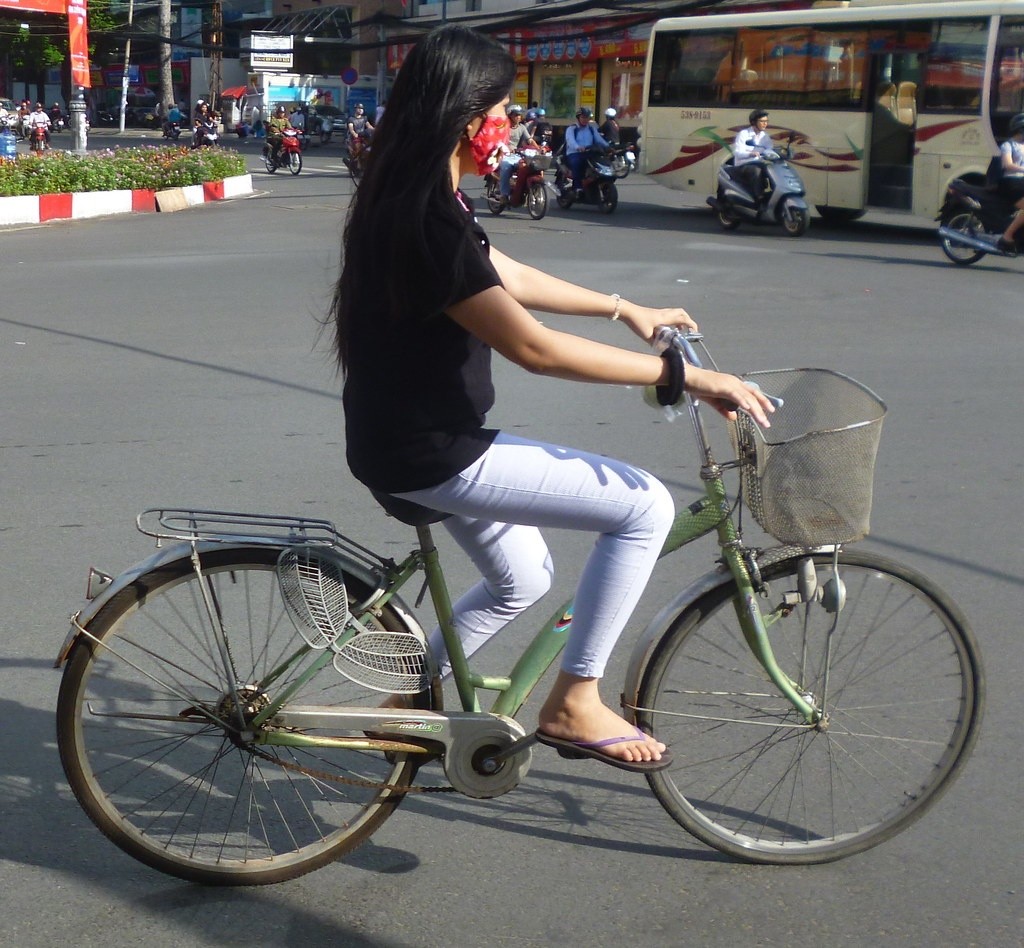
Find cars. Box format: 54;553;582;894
308;105;349;134
0;97;19;129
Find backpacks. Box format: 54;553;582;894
986;140;1015;185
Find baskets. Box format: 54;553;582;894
731;367;889;547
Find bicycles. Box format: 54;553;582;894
51;322;985;887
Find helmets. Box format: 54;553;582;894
168;103;172;108
576;106;592;117
535;109;546;115
605;108;616;116
354;103;364;109
749;109;769;122
1009;113;1024;131
506;105;522;115
36;103;43;108
277;106;286;113
526;109;537;119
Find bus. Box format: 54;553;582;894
638;0;1024;225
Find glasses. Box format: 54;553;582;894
757;119;769;123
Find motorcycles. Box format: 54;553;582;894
480;129;553;220
158;116;182;141
258;114;335;176
545;142;621;216
343;129;376;178
935;178;1024;267
0;114;64;151
613;141;636;179
190;117;219;148
706;130;810;237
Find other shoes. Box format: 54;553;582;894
500;196;510;204
997;237;1016;251
576;189;582;192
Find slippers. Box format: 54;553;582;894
534;724;674;773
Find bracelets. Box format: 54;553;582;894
611;293;621;322
656;347;686;406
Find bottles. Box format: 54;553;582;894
0;128;16;156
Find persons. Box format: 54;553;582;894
500;102;552;206
589;108;620;145
0;95;63;150
734;109;793;210
151;99;185;137
334;27;776;772
566;106;615;195
999;114;1024;252
235;118;270;139
345;100;388;162
193;99;222;149
269;105;334;162
